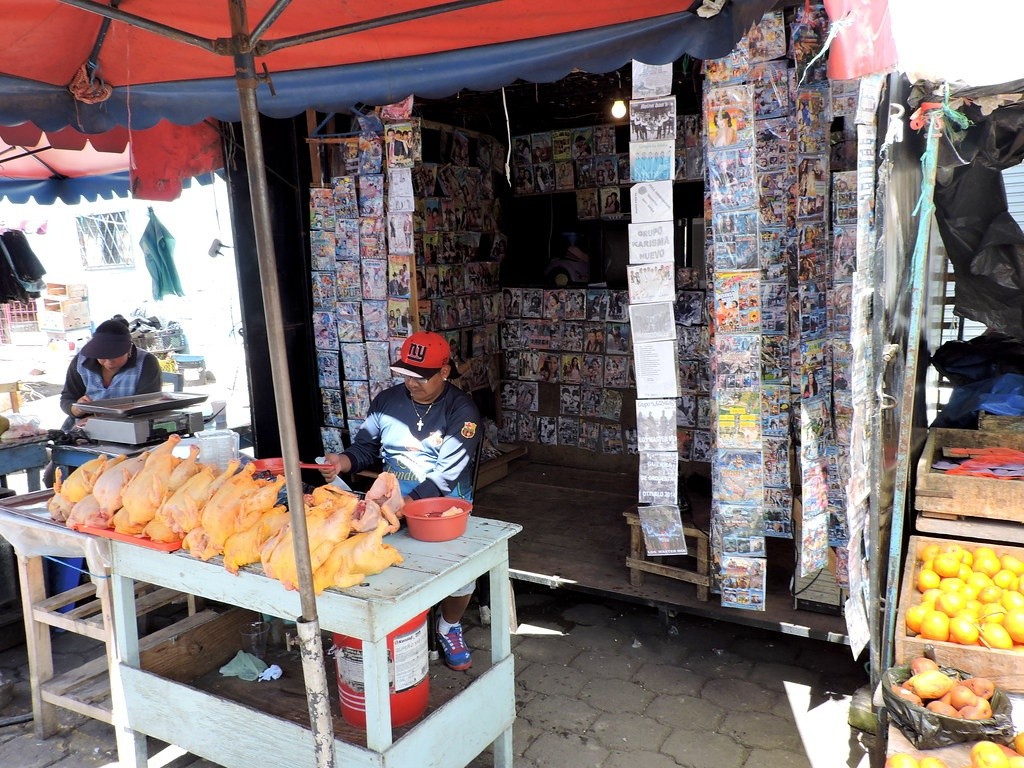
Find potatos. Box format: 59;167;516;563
892;657;994;721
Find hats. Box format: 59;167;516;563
390;331;450;379
834;228;842;236
804;101;809;106
81;333;131;359
749;562;763;572
781;404;788;409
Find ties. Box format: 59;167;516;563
388;144;393;166
399;319;400;324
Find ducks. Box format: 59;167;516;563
47;434;404;595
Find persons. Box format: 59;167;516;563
418;137;501;461
631;20;881;609
498;288;638;458
309;125;415;463
318;332;480;670
510;127;630;220
43;315;162;488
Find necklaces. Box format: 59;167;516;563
411;392;432;432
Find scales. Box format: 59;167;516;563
70;391;210;450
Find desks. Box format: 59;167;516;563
50;413;255;484
0;478;524;768
0;434;50;501
623;475;710;602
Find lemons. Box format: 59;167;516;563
886;732;1024;767
906;544;1024;653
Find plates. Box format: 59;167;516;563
172;438;200;460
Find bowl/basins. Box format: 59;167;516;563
882;665;1012;740
401;497;473;541
172;354;205;362
194;429;239;477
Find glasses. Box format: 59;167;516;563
400;369;440;383
515;143;521;147
576;144;585;149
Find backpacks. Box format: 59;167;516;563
521;139;530;154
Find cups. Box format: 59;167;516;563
238;621;270;660
212;400;226;422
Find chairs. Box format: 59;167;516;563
429;414;491;662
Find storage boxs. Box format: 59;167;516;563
135;333;187;356
39;282;93;358
895;426;1024;695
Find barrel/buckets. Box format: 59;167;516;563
333;606;429;728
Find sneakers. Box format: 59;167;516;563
436;616;472;670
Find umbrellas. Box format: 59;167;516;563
0;1;779;768
